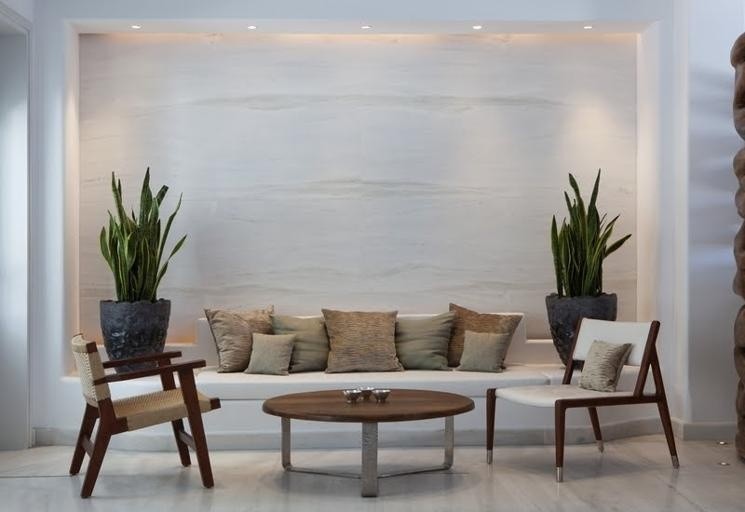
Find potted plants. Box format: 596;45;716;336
99;167;187;372
545;167;633;370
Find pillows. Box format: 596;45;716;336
321;308;404;373
395;310;457;371
455;330;509;372
202;304;274;372
578;339;633;392
270;314;328;373
243;332;295;375
448;302;522;372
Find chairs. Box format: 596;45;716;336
486;315;680;481
69;332;221;498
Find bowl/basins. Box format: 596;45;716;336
358;387;373;400
373;389;390;402
342;389;361;401
